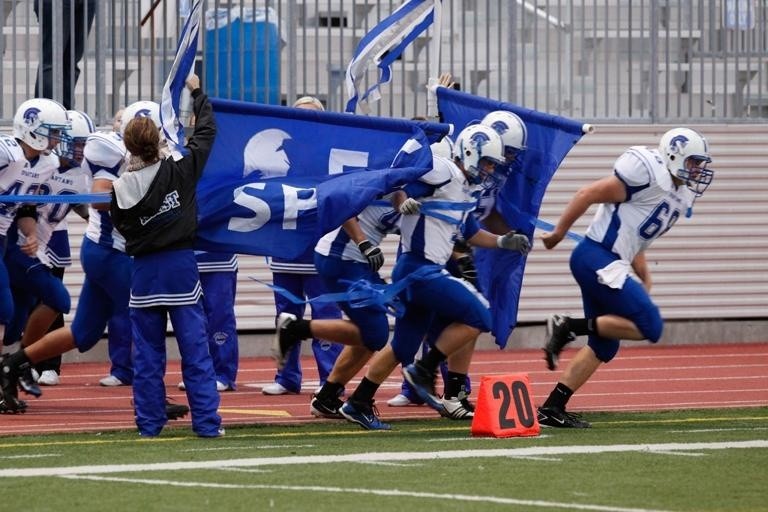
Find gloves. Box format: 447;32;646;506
357;239;384;273
456;256;478;286
498;231;531;255
401;199;421;215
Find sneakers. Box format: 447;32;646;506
262;383;301;395
541;314;576;371
178;382;185;391
98;376;123;386
218;429;225;435
314;385;344;397
537;407;592;428
400;359;445;411
0;353;28;414
216;380;236;391
270;312;297;371
441;395;475;420
31;369;40;382
388;394;425;406
17;368;42;397
38;371;60;386
339;397;391;430
131;396;189;421
310;393;345;418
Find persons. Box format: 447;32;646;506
0;98;74;414
174;251;242;395
0;100;162;410
29;311;66;387
437;110;530;424
10;109;96;401
98;311;135;387
535;127;715;428
109;72;228;439
262;97;346;398
272;133;455;421
336;123;493;431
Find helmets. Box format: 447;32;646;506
453;125;506;190
480;111;530;178
431;136;454;162
658;128;714;193
120;101;166;142
13;98;73;157
66;110;95;144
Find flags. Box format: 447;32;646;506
435;85;592;350
192;92;455;264
